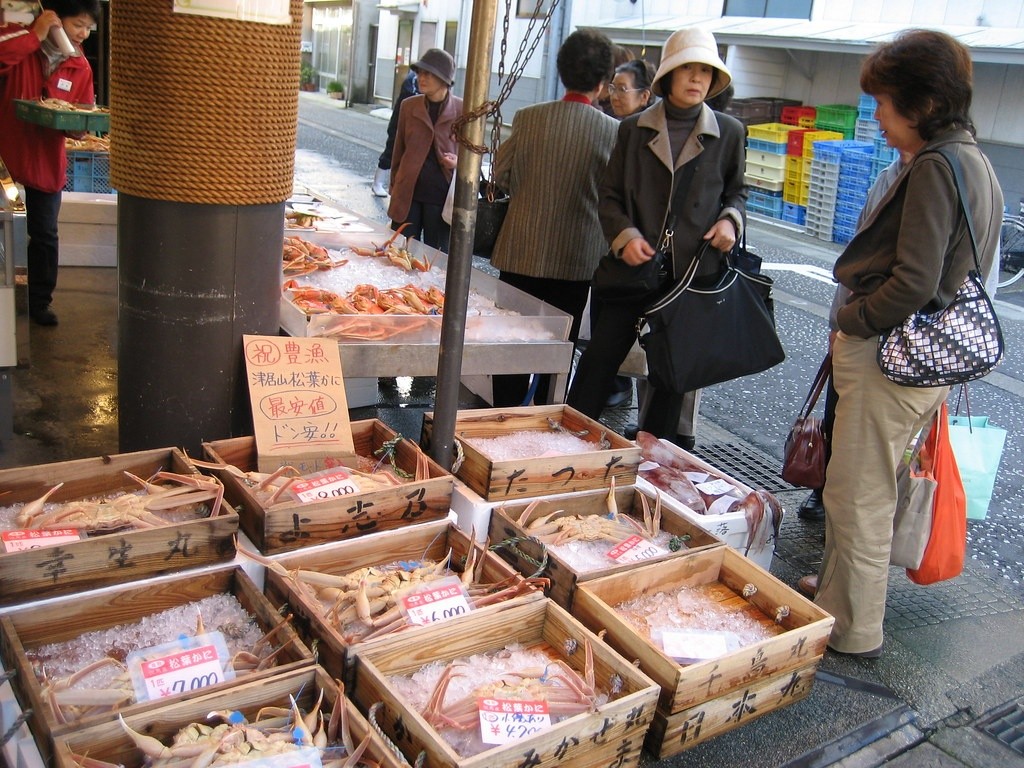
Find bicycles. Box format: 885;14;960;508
995;199;1024;289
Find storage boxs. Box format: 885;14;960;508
17;98;110;131
0;404;836;767
724;94;900;246
0;150;118;267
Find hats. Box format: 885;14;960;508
650;28;732;99
409;49;455;86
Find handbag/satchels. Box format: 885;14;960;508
645;216;785;395
729;206;762;277
472;179;509;259
875;149;1004;387
948;382;1006;521
781;354;832;488
442;169;483;226
888;403;965;584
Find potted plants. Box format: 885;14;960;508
326;81;343;98
300;60;315;91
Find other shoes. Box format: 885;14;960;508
624;423;695;451
603;386;633;409
29;311;57;326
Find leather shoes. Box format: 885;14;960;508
798;492;825;518
825;637;884;657
798;575;818;597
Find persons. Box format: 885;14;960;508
490;25;748;446
370;49;465;395
0;0;100;328
799;33;1005;663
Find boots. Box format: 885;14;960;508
372;166;388;197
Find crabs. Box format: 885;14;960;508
41;522;597;768
518;469;664;548
34;94;111;153
15;440;437;538
281;210;447;341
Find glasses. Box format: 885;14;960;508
608;84;643;97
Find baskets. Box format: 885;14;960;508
746;122;805;154
804;139;874;246
745;190;783;221
854;96;882;142
722;97;802;156
